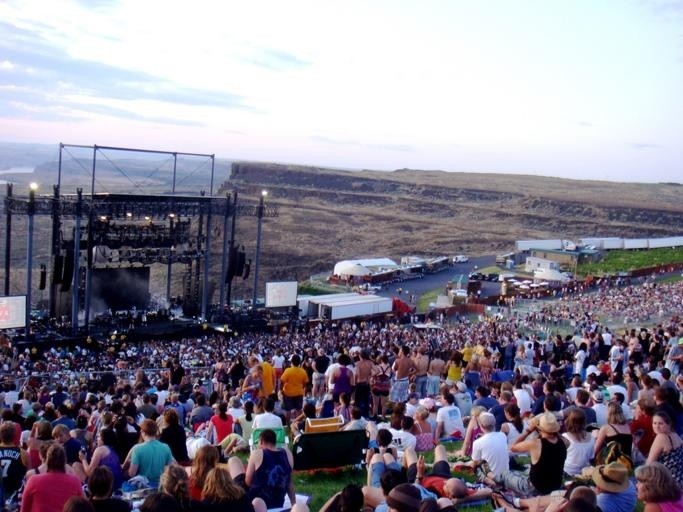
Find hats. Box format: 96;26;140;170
479;410;633;492
385;483;422;512
139;419;157;432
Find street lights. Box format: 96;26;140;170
24;181;40;338
250;190;267;313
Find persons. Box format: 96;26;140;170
495;269;681;326
0;303;682;512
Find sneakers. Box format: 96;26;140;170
473;459;494;484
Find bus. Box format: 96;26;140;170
495;252;515;264
422;256;449;274
362;265;424;289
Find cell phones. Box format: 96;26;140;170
81;445;86;455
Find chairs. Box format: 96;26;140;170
304;417;347;432
248;425;288;456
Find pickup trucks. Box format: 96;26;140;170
358;283;382;293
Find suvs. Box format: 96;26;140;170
453;255;468;263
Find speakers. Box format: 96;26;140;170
53;256;64;285
61;245;75;292
40;271;46;290
225;247;237;283
237;252;245;276
243;264;250;280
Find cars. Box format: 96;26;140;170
452;289;467;296
468;272;486;281
